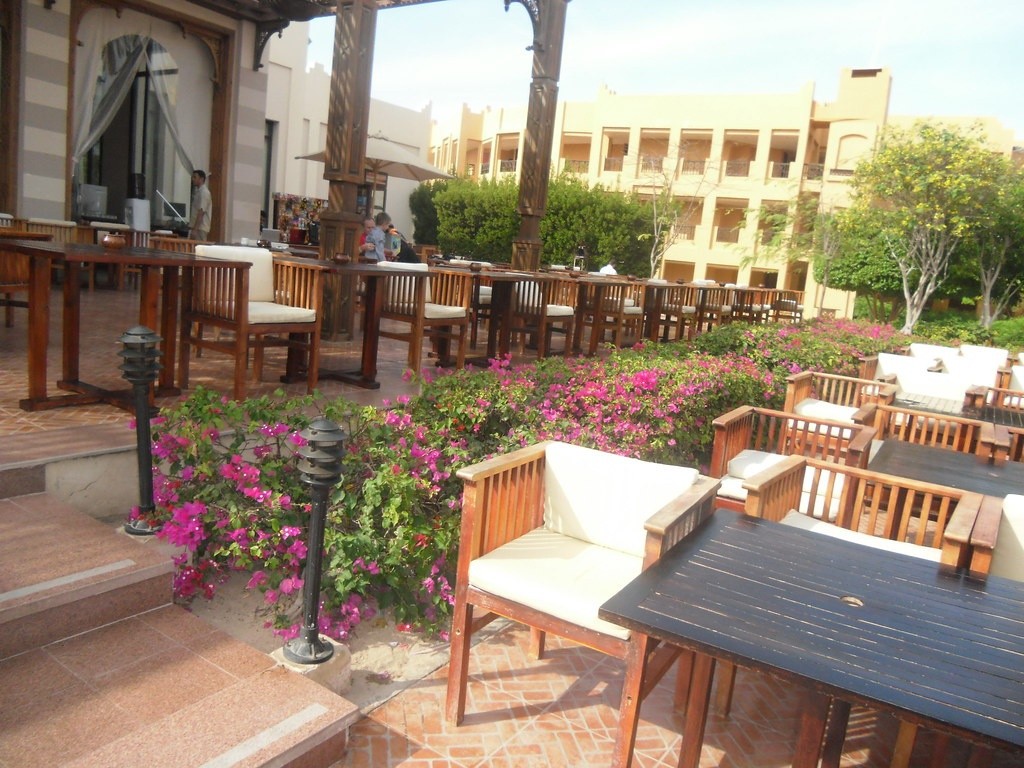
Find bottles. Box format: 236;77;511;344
126;174;145;200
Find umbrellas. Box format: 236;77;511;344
295;139;455;215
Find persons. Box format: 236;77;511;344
260;210;265;231
600;258;618;275
358;212;398;262
188;170;213;241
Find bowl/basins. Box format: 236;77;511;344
103;234;126;249
569;271;580;278
676;279;684;284
334;253;349;265
469;262;482;272
719;282;726;287
627;274;636;282
257;239;271;247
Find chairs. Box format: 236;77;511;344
0;212;808;402
446;342;1024;767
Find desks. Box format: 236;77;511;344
432;267;536;371
980;408;1024;462
548;272;634;359
276;256;441;390
863;438;1024;544
698;286;724;332
735;288;760;323
638;281;688;342
597;509;1024;768
0;236;253;420
